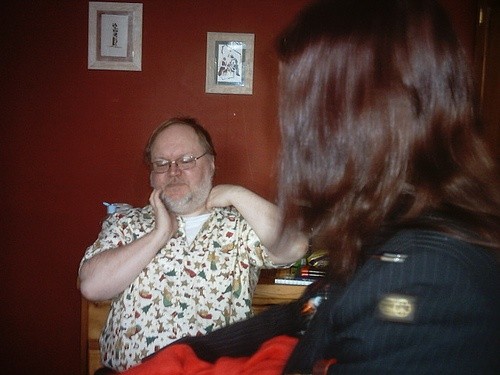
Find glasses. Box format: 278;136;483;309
150;150;209;174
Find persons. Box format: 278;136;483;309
120;1;500;375
78;115;309;375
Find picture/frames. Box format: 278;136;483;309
205;32;254;95
88;2;143;71
